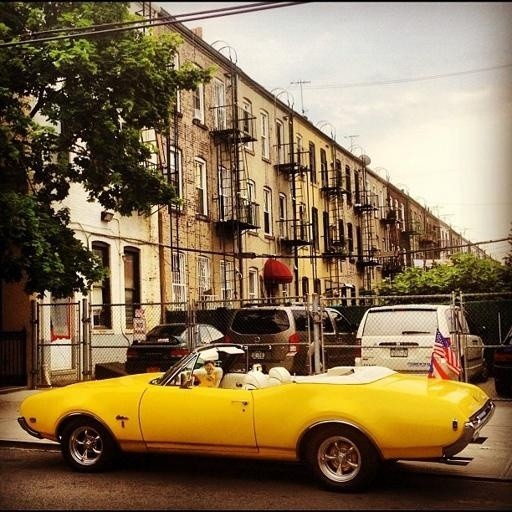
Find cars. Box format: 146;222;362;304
16;343;496;493
489;328;512;397
126;323;225;372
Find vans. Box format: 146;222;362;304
222;301;356;377
353;304;490;384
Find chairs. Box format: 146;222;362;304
269;366;293;385
243;371;269;389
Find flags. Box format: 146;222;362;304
428;328;463;380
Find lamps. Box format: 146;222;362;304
101;212;114;222
